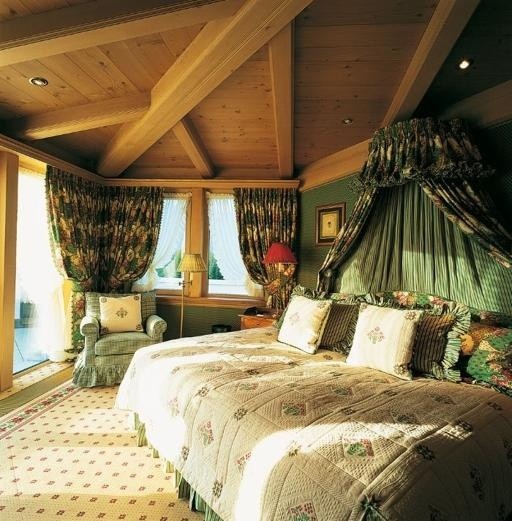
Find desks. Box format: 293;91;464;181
152;289;268;310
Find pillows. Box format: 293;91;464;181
366;292;470;383
378;291;512;399
276;284;359;356
346;302;424;380
99;294;145;335
276;295;333;355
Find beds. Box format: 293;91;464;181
109;291;512;521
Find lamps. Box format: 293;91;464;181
177;254;208;339
262;242;298;319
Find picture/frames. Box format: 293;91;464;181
315;202;345;246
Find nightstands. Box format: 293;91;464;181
237;312;276;331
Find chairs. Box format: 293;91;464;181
72;294;167;388
14;302;37;362
